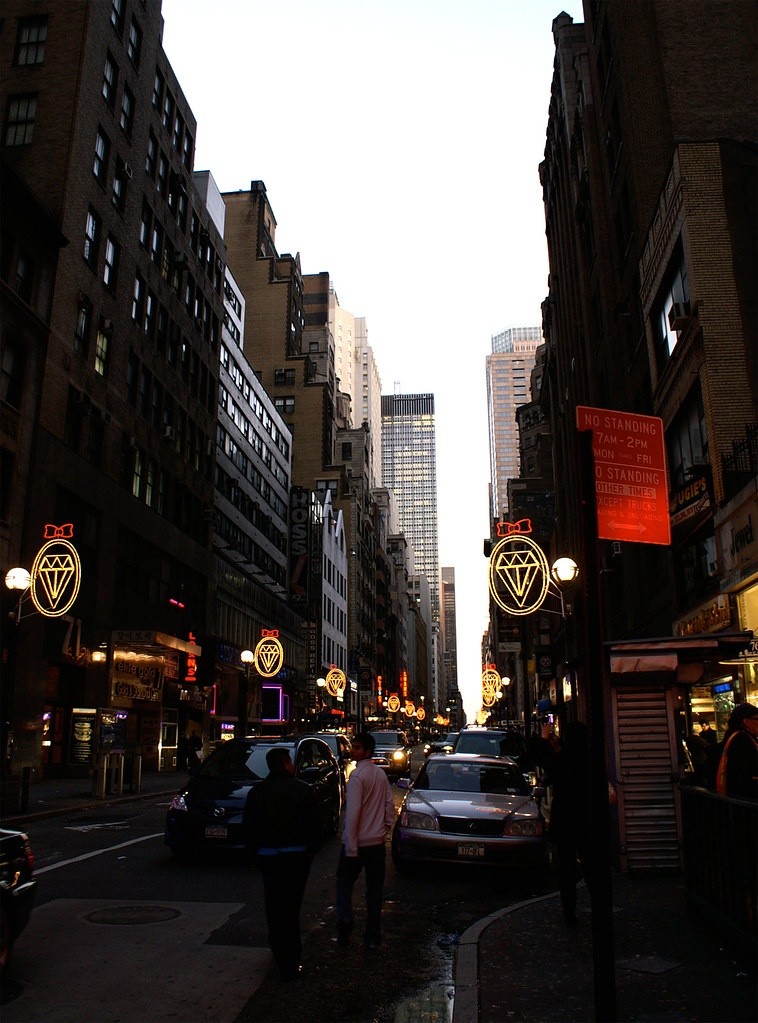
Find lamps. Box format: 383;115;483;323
316;678;326;689
240;650;255;678
446;708;451;711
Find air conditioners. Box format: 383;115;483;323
164;425;175;441
667;302;689;330
122;161;133;180
196;318;203;330
105;319;115;334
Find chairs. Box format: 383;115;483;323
478;768;516;794
430;764;462;790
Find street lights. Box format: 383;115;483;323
549;554;583;746
240;649;255;698
317;677;325;705
4;565;32;686
382;696;495;725
501;676;511;724
496;691;504;722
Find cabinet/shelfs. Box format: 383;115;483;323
139;706;180;774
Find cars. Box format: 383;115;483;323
300;724;538;821
0;827;37;973
162;734;342;868
389;751;543;883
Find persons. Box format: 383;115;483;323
533;718;606;926
336;733;395;948
243;748;333;983
678;703;758;928
178;729;204;767
